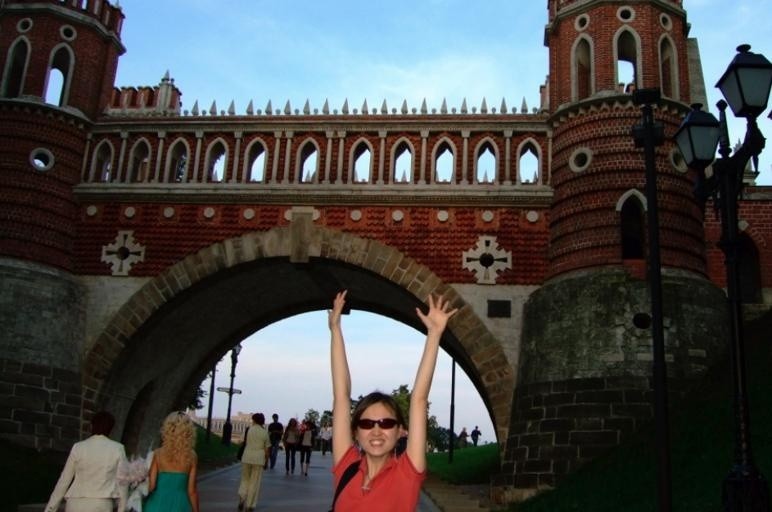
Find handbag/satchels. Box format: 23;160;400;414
237;428;249;460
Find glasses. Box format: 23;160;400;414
358;418;397;429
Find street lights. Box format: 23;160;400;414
224;342;242;444
670;45;772;512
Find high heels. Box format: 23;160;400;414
239;503;256;512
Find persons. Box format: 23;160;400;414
318;290;458;512
237;414;332;512
458;426;481;447
45;410;199;512
